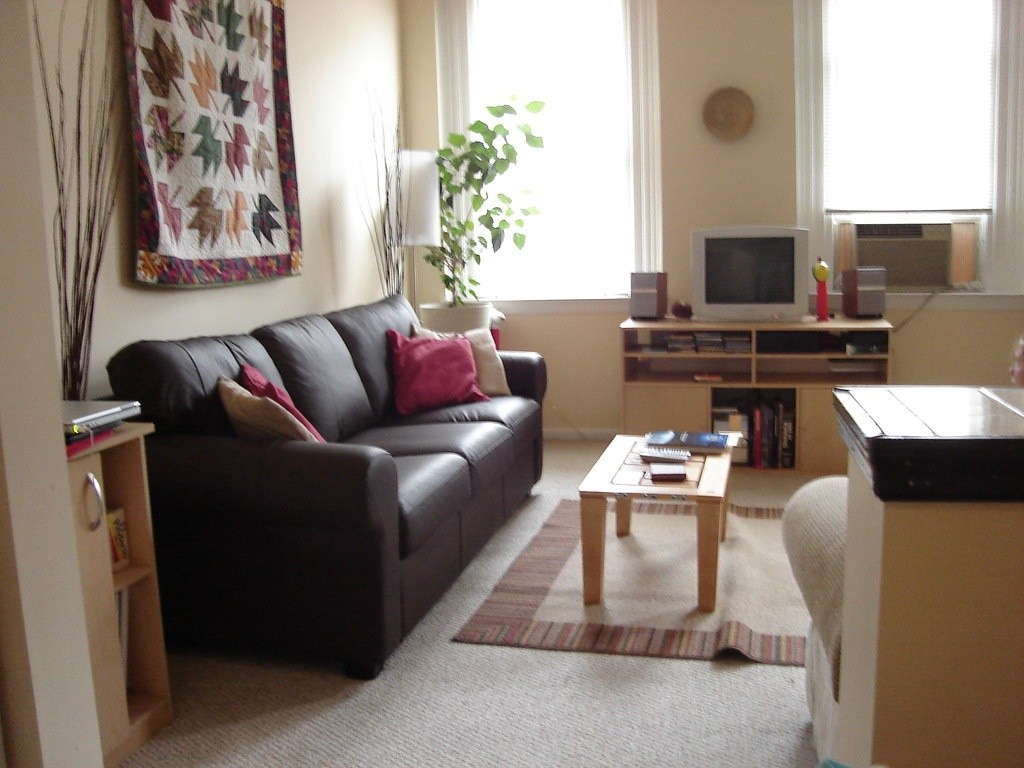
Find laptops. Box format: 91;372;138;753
62;400;142;434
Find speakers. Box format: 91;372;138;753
630;272;668;319
842;267;887;318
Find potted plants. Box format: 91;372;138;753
418;99;547;336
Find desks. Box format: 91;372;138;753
577;431;736;611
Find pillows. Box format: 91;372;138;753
410;323;512;397
218;375;317;444
240;359;330;444
385;325;490;419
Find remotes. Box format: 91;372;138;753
638;446;691;464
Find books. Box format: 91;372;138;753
112;590;130;687
639;429;728;482
712;392;795;469
724;336;750;353
663;334;695;352
694;332;724;352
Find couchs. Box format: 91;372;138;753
105;289;548;684
779;470;849;761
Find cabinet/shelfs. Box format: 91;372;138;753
831;382;1023;768
67;417;176;768
616;309;894;471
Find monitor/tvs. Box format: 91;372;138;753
689;226;811;321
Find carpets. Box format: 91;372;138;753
455;497;809;668
